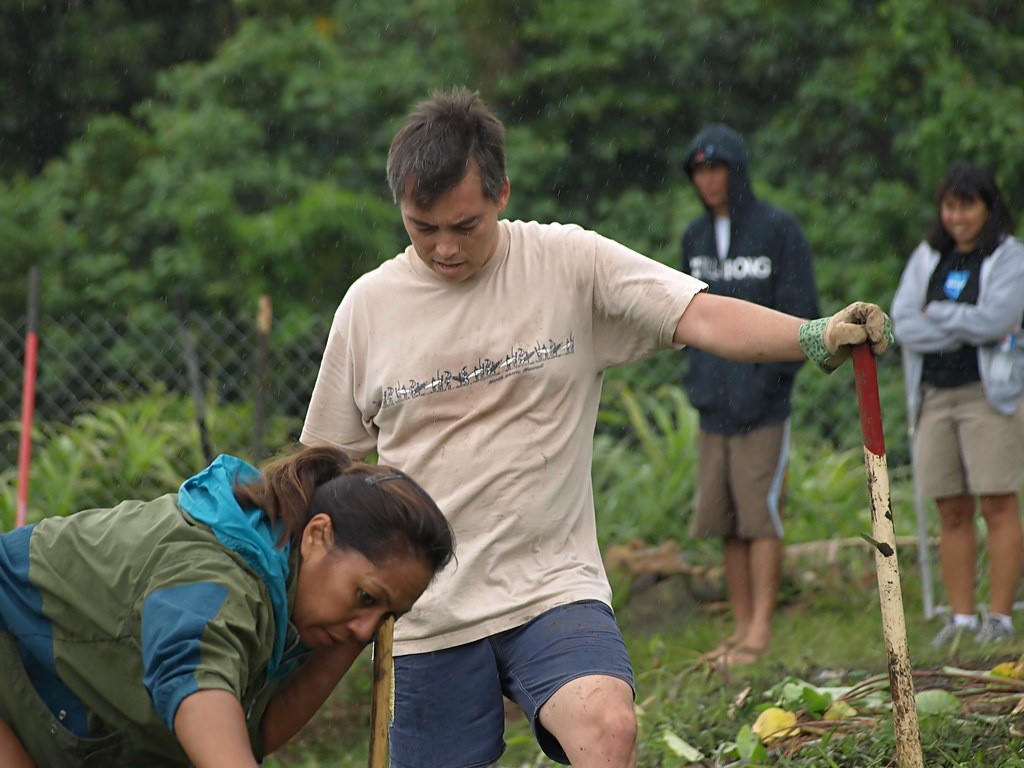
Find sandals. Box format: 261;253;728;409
696;638;774;671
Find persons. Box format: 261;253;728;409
680;121;820;670
0;447;454;768
298;91;891;768
889;164;1024;647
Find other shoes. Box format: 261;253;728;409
972;613;1015;648
930;613;982;651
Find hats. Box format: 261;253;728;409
690;150;727;166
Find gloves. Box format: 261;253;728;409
798;301;894;376
